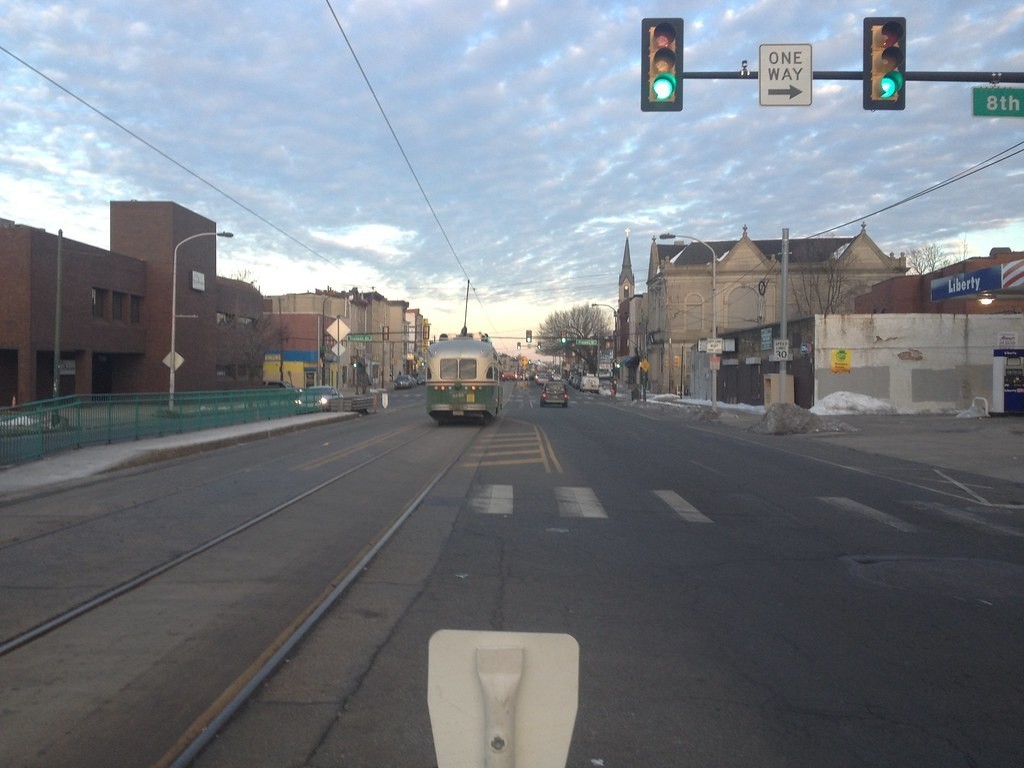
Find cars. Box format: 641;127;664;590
294;386;344;413
540;380;568;407
501;370;560;383
394;374;425;389
264;381;303;398
568;374;599;394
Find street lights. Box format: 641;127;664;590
659;233;718;414
591;302;616;330
322;291;354;386
167;231;233;410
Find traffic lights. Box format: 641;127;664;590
538;341;542;350
560;338;568;344
862;17;905;110
642;18;683;111
516;342;521;349
525;329;532;343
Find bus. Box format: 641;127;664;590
425;280;503;423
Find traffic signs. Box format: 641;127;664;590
758;43;812;106
